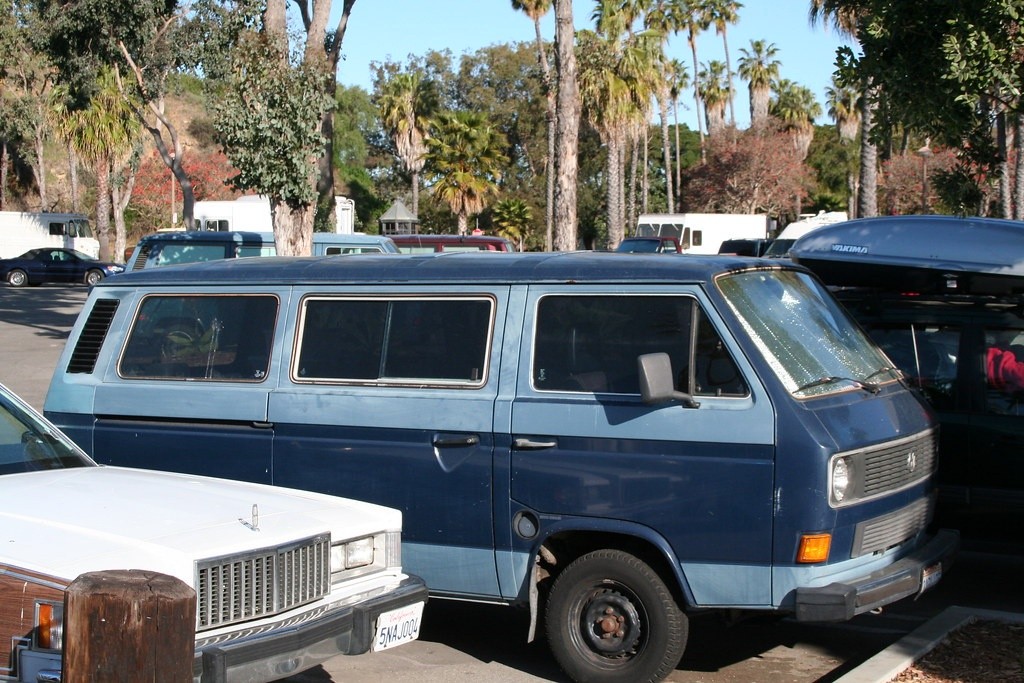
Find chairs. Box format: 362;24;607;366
54;255;60;260
48;255;52;260
568;366;632;392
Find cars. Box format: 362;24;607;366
0;246;132;289
781;216;1023;562
0;379;432;683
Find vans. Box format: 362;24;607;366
118;229;404;276
1;210;101;258
32;251;960;683
344;237;518;272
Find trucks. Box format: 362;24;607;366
760;209;849;261
614;213;773;253
